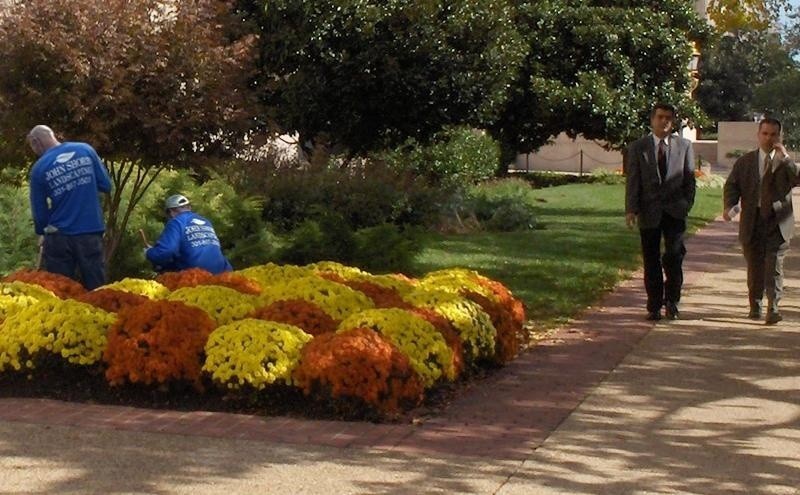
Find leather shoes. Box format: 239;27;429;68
749;309;782;323
646;301;679;320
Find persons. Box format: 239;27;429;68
27;123;113;292
142;192;233;277
623;101;697;320
723;116;799;324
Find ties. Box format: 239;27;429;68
760;154;772;221
658;140;667;182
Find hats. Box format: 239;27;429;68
165;194;189;209
